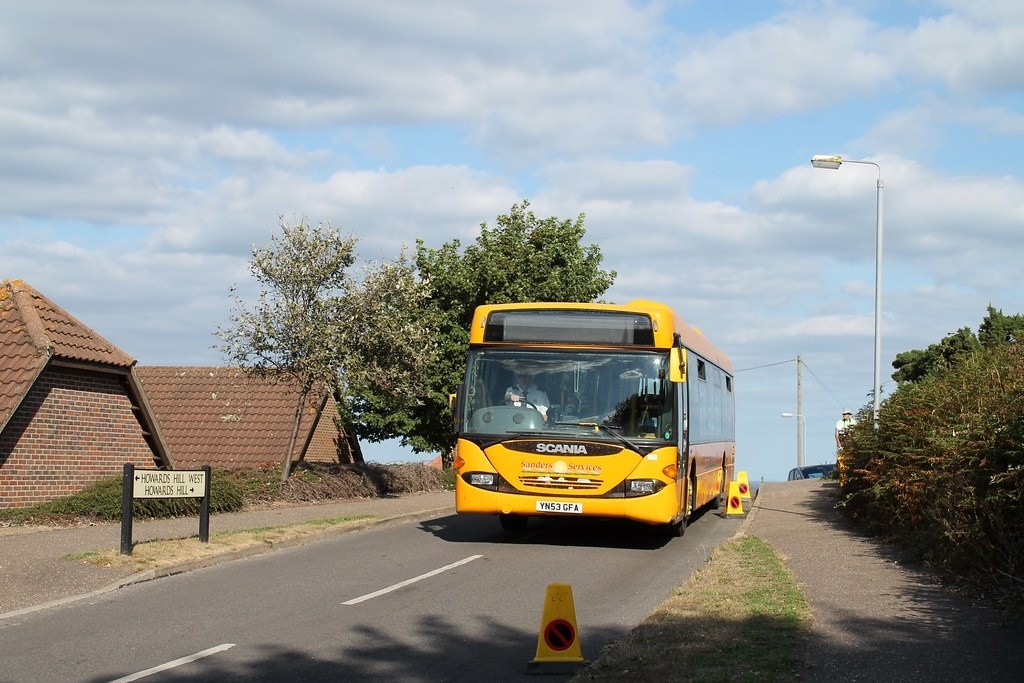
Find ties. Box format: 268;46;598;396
521;391;529;408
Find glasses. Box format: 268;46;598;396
517;374;527;379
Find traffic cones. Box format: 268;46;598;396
718;480;748;520
524;582;594;678
736;470;752;502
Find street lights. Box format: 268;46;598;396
783;411;807;468
810;150;885;435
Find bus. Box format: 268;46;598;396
445;297;735;537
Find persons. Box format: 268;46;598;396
505;366;550;423
547;372;655;428
835;408;859;486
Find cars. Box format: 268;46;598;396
788;464;839;482
